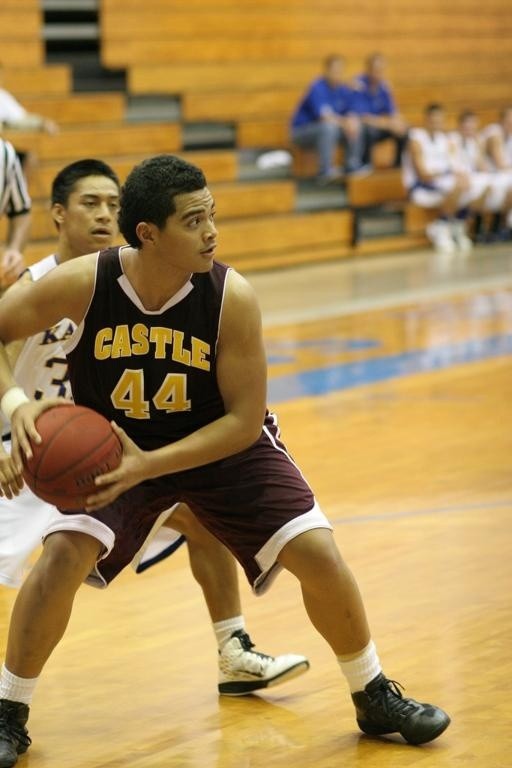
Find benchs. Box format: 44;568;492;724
0;0;512;291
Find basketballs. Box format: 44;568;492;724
18;404;121;511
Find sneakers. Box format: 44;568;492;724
217;633;311;697
0;698;30;767
350;671;450;746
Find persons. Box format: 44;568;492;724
1;136;34;281
289;51;511;254
0;155;451;768
1;160;309;696
